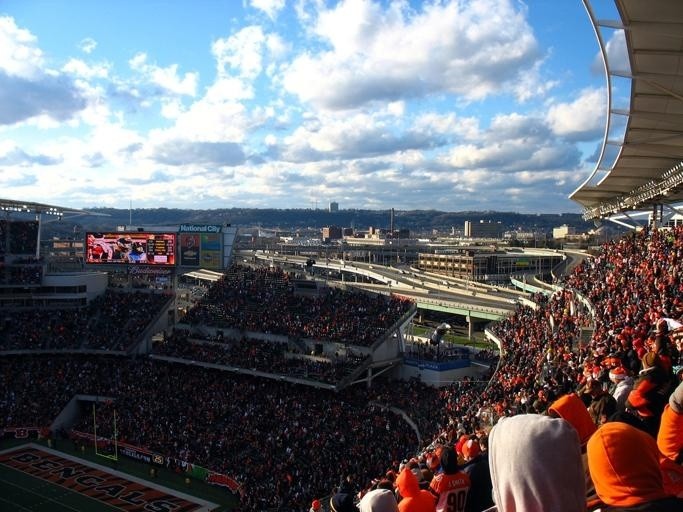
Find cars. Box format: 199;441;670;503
470;282;478;287
492;287;499;292
439;281;459;288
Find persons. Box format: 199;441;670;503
0;219;683;512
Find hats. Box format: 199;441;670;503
312;500;320;508
643;352;661;368
608;367;625;380
462;440;480;457
329;493;354;512
455;437;468;452
